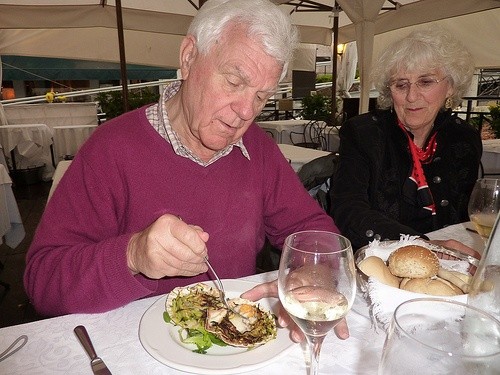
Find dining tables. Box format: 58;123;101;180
0;223;485;375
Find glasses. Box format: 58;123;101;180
387;76;447;94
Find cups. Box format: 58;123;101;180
460;214;500;358
377;299;500;375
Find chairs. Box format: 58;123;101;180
266;111;347;209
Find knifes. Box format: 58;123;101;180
74;326;112;375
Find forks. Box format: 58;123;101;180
178;216;238;315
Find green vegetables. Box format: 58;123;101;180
162;286;276;354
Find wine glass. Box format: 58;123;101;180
278;230;357;374
468;178;500;244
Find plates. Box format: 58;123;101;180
139;278;299;374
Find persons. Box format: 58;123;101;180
23;0;349;344
333;30;483;259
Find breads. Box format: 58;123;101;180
357;244;494;297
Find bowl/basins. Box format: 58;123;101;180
353;239;480;297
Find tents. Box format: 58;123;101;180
0;0;500;112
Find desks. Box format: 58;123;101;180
0;124;56;171
276;141;341;198
52;125;99;161
257;120;326;145
